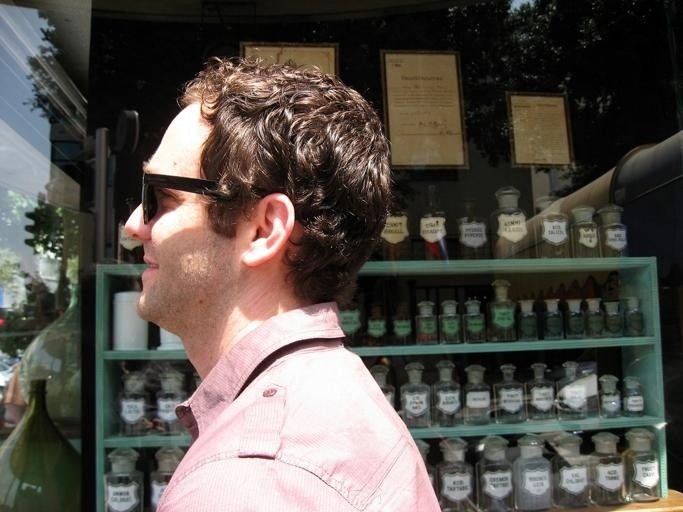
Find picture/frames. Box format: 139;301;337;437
379;49;470;170
238;40;340;82
504;90;577;170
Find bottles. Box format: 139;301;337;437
384;184;627;260
415;429;660;512
371;357;650;424
116;363;192;437
341;277;650;350
103;446;183;509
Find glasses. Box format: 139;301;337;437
142;171;300;224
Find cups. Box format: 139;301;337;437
111;291;148;350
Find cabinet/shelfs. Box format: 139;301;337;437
94;255;669;512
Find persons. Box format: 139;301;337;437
0;272;75;430
122;52;440;511
